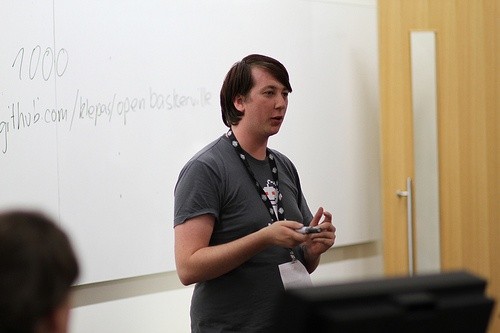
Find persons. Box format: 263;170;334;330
0;211;79;333
172;54;335;333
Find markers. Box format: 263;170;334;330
293;226;321;235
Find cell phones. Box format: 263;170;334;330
297;226;320;234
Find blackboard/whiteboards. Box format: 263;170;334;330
0;0;379;294
